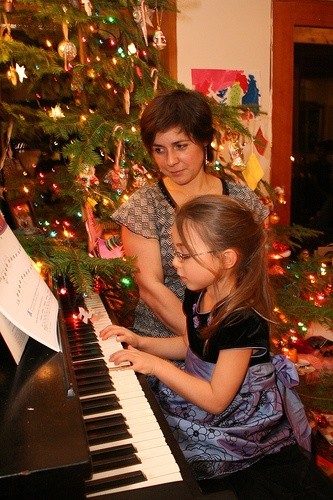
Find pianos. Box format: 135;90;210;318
0;242;206;500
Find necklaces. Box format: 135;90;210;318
166;177;212;199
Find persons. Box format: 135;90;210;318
100;195;313;481
110;91;272;388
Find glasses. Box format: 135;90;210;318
169;246;220;262
